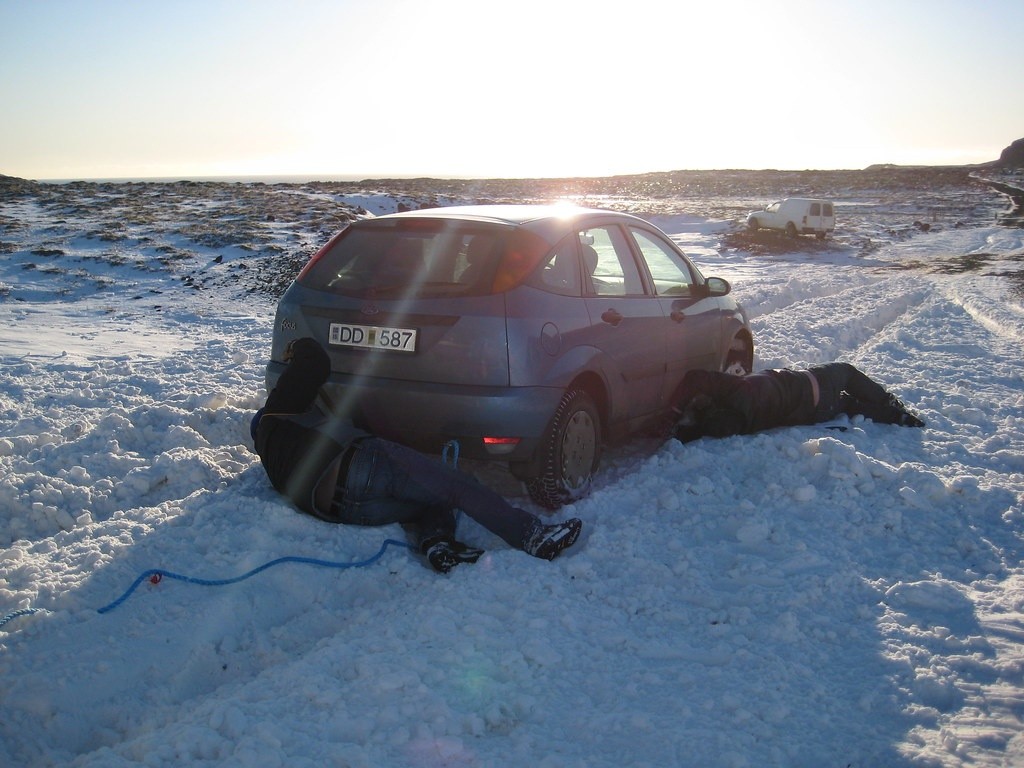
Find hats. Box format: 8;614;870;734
250;409;266;439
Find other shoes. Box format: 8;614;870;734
905;416;924;428
889;392;906;412
523;518;582;559
424;539;484;573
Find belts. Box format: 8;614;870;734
332;437;361;516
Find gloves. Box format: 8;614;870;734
661;407;677;435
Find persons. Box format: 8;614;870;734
663;363;925;444
250;337;581;573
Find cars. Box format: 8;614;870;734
264;201;754;511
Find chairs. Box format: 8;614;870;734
544;244;598;293
370;240;432;279
459;236;495;284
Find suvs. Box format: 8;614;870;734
746;196;836;241
